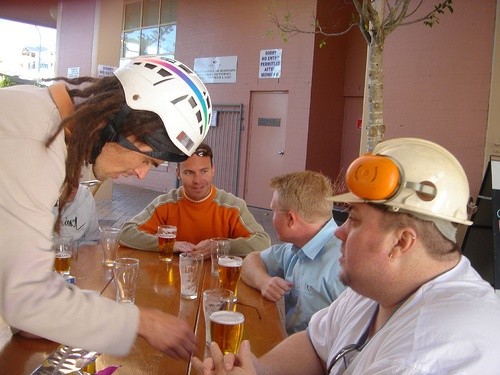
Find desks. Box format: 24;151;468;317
0;245;285;375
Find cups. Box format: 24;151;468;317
113;258;140;304
218;255;242;303
202;288;234;347
209;310;245;355
179;252;203;300
100;227;123;266
158;225;177;262
209;237;231;277
54;236;78;283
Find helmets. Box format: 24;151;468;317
325;137;473;226
113;54;213;157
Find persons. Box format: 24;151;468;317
116;143;271;262
192;136;500;375
53;185;102;246
240;170;348;336
0;57;213;362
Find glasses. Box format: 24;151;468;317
191;149;212;166
327;343;360;375
